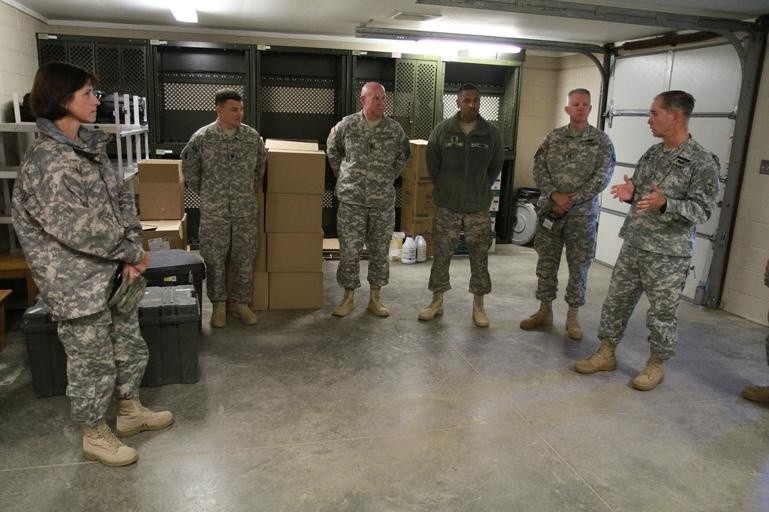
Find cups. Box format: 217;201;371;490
161;288;178;304
138;290;153;307
160;240;171;257
148;238;162;254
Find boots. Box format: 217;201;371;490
521;301;555;330
565;305;583;340
211;301;226;328
230;302;258;324
333;289;356;317
418;292;443;320
742;385;769;404
574;340;617;373
367;288;390;316
633;354;663;390
115;397;174;437
472;294;489;326
83;419;138;467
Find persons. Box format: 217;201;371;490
520;88;616;341
572;90;721;392
10;62;174;468
181;90;267;329
326;81;412;319
418;84;503;327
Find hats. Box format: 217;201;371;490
109;271;148;323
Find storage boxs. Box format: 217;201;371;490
145;249;206;332
227;192;324;312
401;178;437;256
265;140;319;151
401;139;432;183
138;174;185;220
19;284;202;399
267;149;325;194
137;160;183;183
139;213;190;252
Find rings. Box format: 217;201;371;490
135;272;139;277
